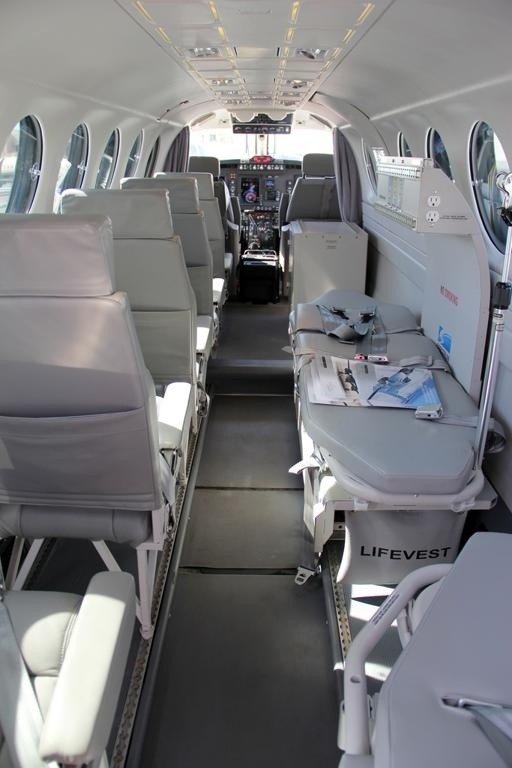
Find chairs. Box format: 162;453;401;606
277;153;344;297
0;155;243;638
1;570;143;768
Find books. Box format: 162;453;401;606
308;349;444;411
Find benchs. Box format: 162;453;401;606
290;289;497;590
